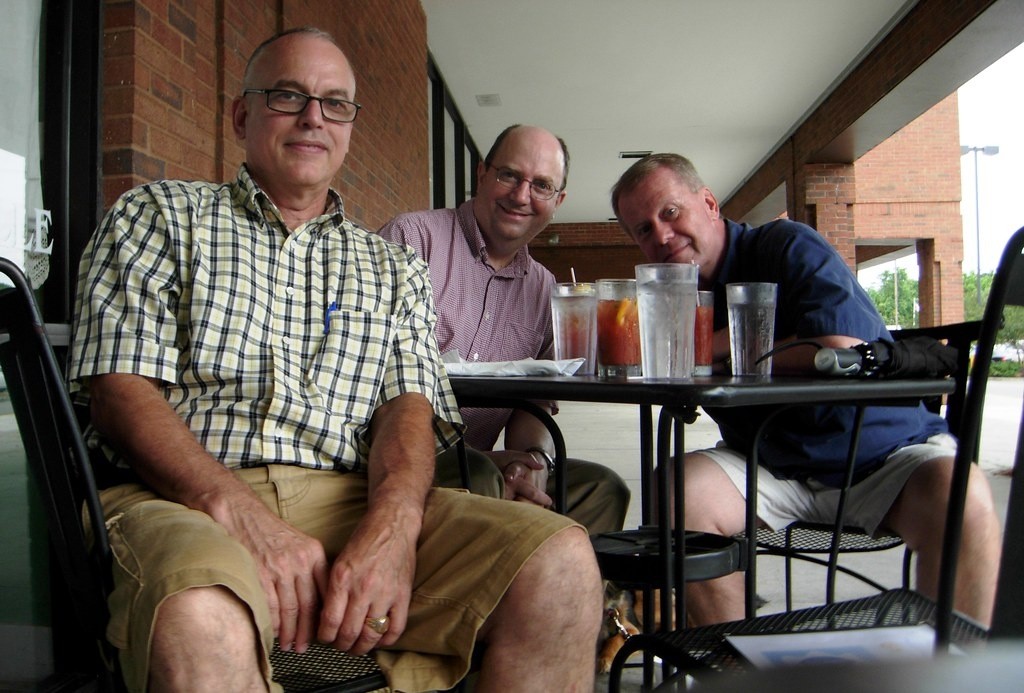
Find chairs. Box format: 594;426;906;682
0;258;388;693
608;228;1024;693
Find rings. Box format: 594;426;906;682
365;617;390;634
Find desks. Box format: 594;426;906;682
449;372;957;693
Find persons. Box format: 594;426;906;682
375;124;630;548
613;152;1000;631
65;26;605;692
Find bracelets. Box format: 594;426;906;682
527;446;556;476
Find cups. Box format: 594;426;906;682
634;263;699;378
595;278;641;378
725;282;778;376
549;283;598;376
694;290;715;377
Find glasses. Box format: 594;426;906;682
483;160;561;200
244;87;363;124
